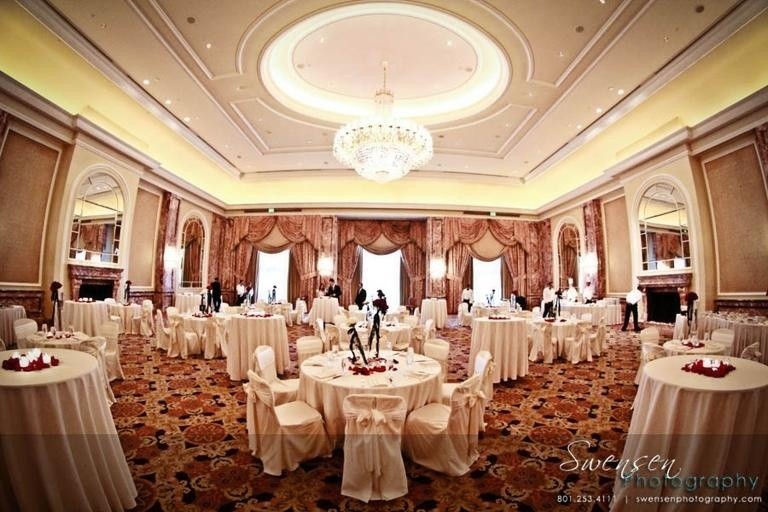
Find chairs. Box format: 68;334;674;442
341;394;410;503
609;307;767;512
441;350;496;435
241;370;334;478
251;344;300;405
131;285;628;384
402;372;482;478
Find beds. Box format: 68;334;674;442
1;289;137;512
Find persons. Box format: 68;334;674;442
209;276;223;312
542;281;556;318
236;279;247;306
583;281;595;304
354;282;367;310
620;285;644;331
315;283;328;298
461;283;476;312
567;284;578;302
244;281;254;305
324;278;343;303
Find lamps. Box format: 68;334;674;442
330;58;436;186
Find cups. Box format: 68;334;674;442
677;331;710;348
326;341;414;377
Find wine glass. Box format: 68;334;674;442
40;323;74;340
